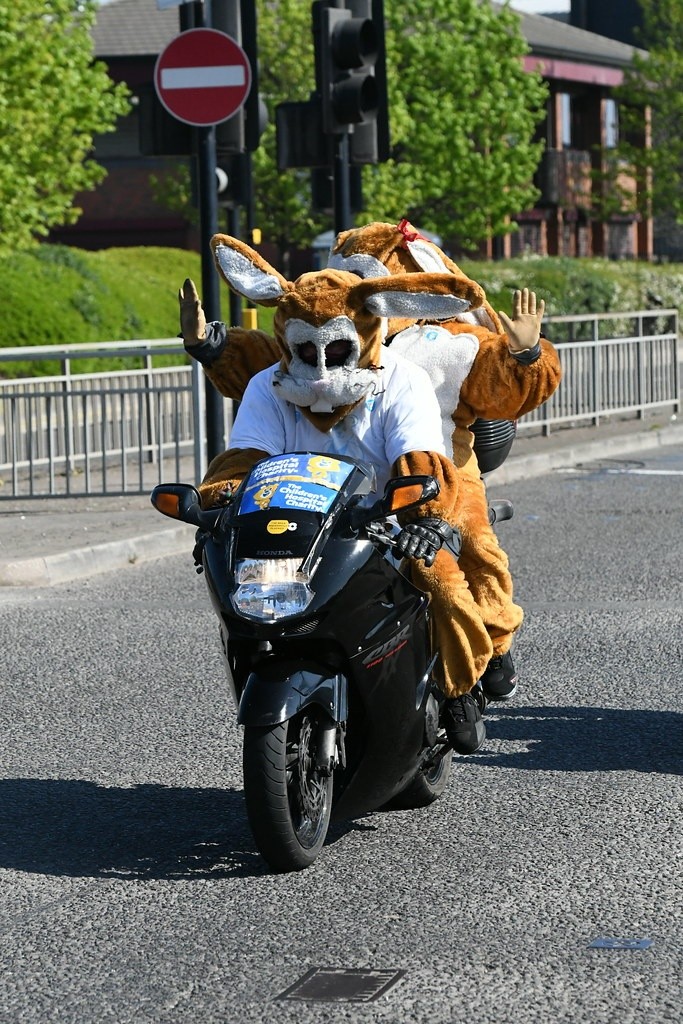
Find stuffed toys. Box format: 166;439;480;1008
170;233;498;759
177;219;570;702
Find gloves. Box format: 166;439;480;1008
391;517;453;567
178;278;206;345
498;288;545;355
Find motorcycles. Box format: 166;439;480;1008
151;450;489;870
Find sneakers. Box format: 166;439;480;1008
441;692;487;754
481;651;517;701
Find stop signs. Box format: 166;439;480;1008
152;26;252;127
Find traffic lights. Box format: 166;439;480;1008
322;6;380;136
